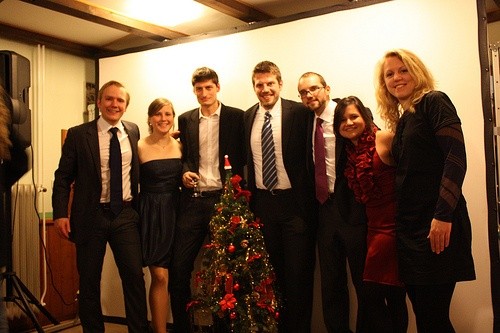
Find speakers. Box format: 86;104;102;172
0;50;31;148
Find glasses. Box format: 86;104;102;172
297;86;326;99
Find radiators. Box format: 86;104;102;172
6;184;40;321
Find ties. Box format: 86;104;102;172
261;112;279;191
314;118;330;204
109;128;123;218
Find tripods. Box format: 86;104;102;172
0;148;61;333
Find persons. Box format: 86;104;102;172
52;80;181;333
375;50;477;333
137;98;184;333
332;95;409;333
170;66;247;333
245;61;374;333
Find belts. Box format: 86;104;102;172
99;201;131;209
258;188;293;196
192;191;220;198
